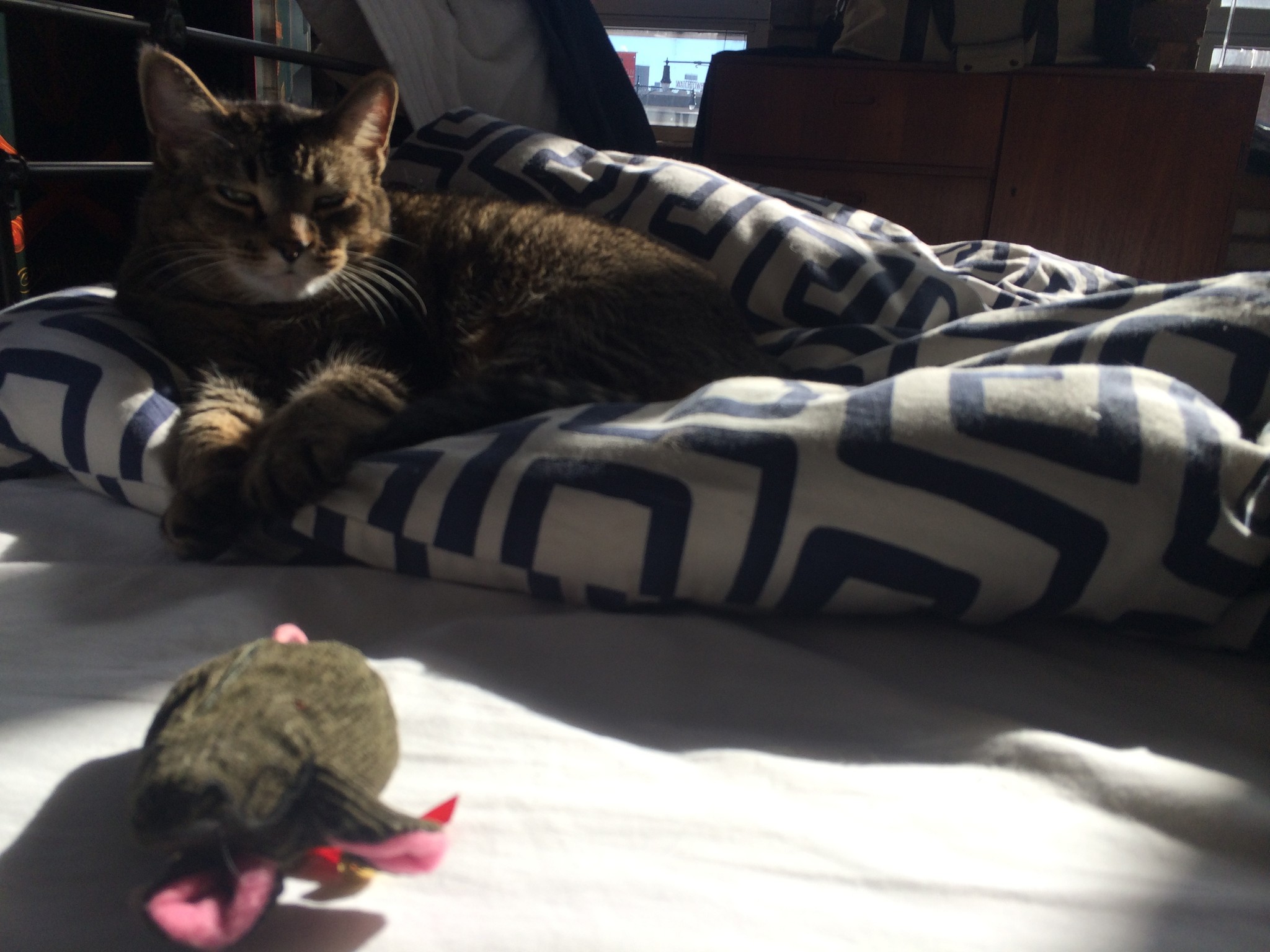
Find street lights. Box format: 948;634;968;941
633;57;710;111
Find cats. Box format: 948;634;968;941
112;40;782;565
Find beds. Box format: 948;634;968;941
0;0;1270;951
